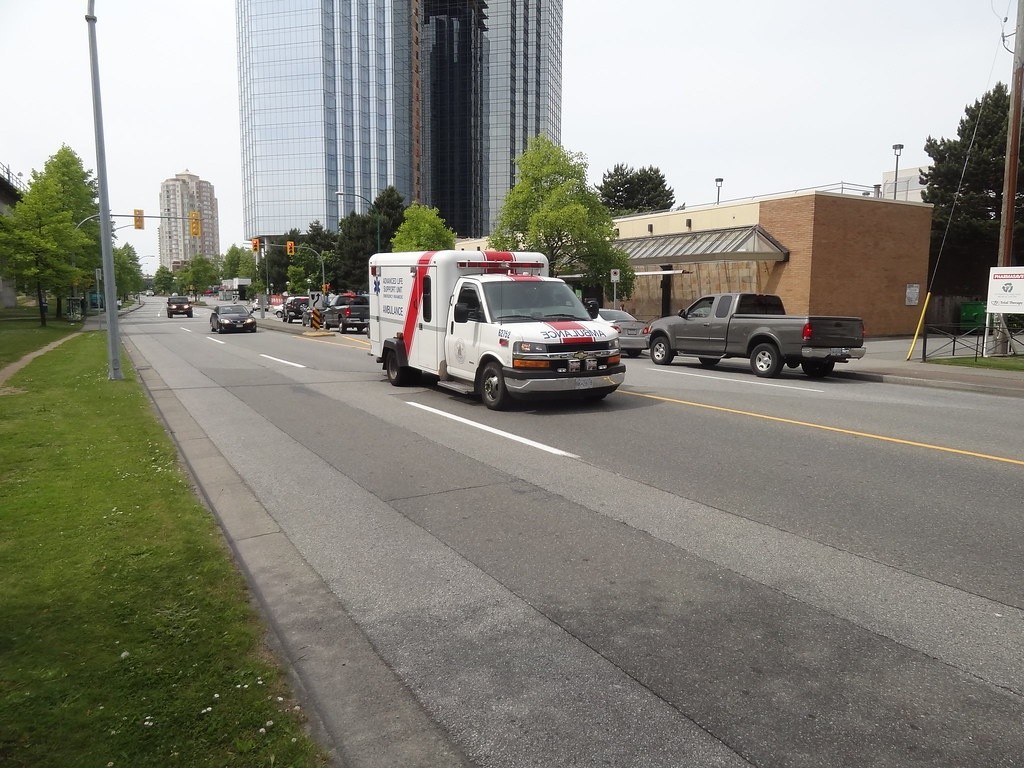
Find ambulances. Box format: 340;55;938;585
365;250;626;411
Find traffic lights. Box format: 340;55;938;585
252;239;259;252
287;242;294;255
320;284;330;295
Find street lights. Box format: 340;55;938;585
245;246;270;297
307;279;311;295
270;283;273;297
335;191;380;253
715;177;723;205
893;144;904;199
137;255;155;305
286;281;290;297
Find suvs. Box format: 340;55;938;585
167;296;193;318
146;290;154;296
282;297;309;323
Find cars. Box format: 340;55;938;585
273;303;284;318
89;294;102;308
591;307;650;357
252;299;269;312
172;293;178;296
210;305;258;334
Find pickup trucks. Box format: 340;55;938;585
322;294;371;334
645;294;866;380
